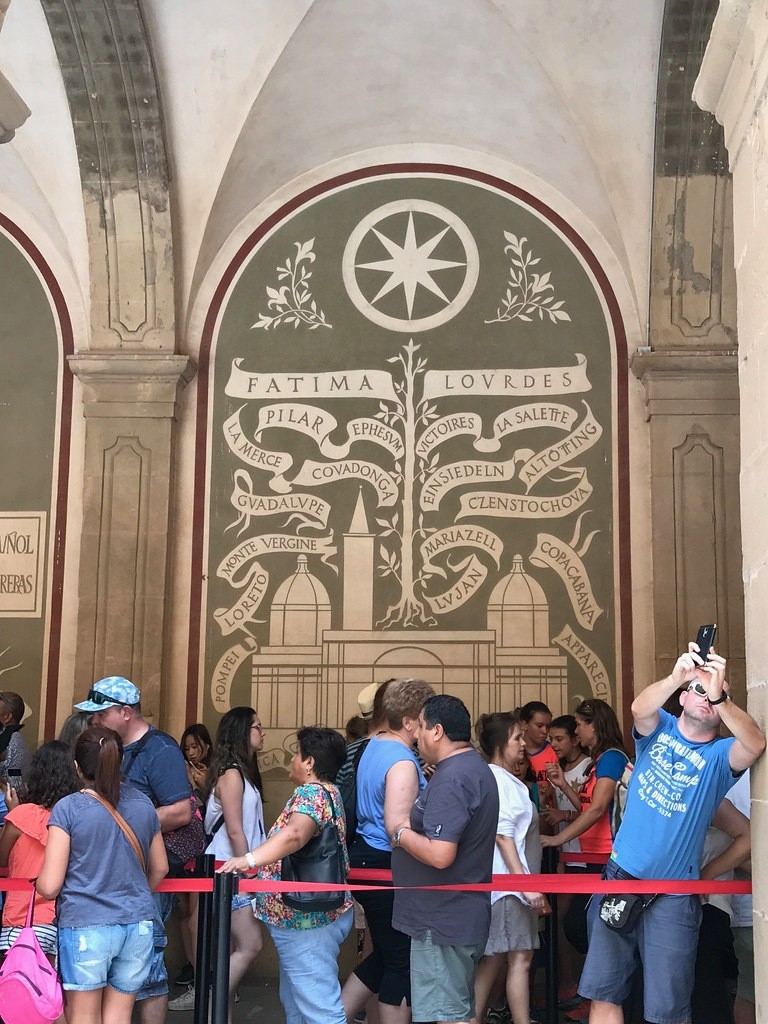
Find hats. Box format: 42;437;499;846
74;676;140;712
358;683;384;720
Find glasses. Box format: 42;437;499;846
88;689;131;706
251;725;263;731
687;682;708;697
581;699;595;714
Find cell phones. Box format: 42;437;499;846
189;761;198;771
693;624;717;667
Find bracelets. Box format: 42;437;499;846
568;810;571;822
245;853;257;870
708;689;727;705
566;810;568;823
570;810;573;822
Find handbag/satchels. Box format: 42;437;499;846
281;782;344;910
599;894;644;934
162;795;207;866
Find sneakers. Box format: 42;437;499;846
487;1002;537;1024
176;962;195;984
168;985;195;1011
565;999;591;1021
557;980;580;1004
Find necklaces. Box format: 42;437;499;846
564;751;582;763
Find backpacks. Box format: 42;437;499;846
596;749;635;844
0;877;65;1024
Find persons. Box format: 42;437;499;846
577;642;766;1024
335;677;755;1024
0;676;215;1024
204;706;266;1024
214;725;354;1024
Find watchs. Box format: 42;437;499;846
394;827;410;847
